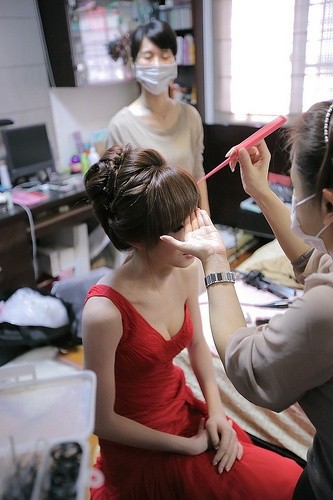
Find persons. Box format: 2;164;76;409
81;144;305;500
107;20;210;217
160;99;333;500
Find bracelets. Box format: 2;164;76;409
204;272;235;288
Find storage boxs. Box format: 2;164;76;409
1;360;97;500
38;224;93;277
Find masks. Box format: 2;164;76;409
289;190;333;255
134;63;179;96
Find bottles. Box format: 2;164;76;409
80;142;100;175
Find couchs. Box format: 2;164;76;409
201;124;291;242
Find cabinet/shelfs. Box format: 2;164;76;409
154;0;206;123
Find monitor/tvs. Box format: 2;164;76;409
0;122;55;178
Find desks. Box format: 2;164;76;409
0;188;94;298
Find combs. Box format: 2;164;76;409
197;114;287;185
